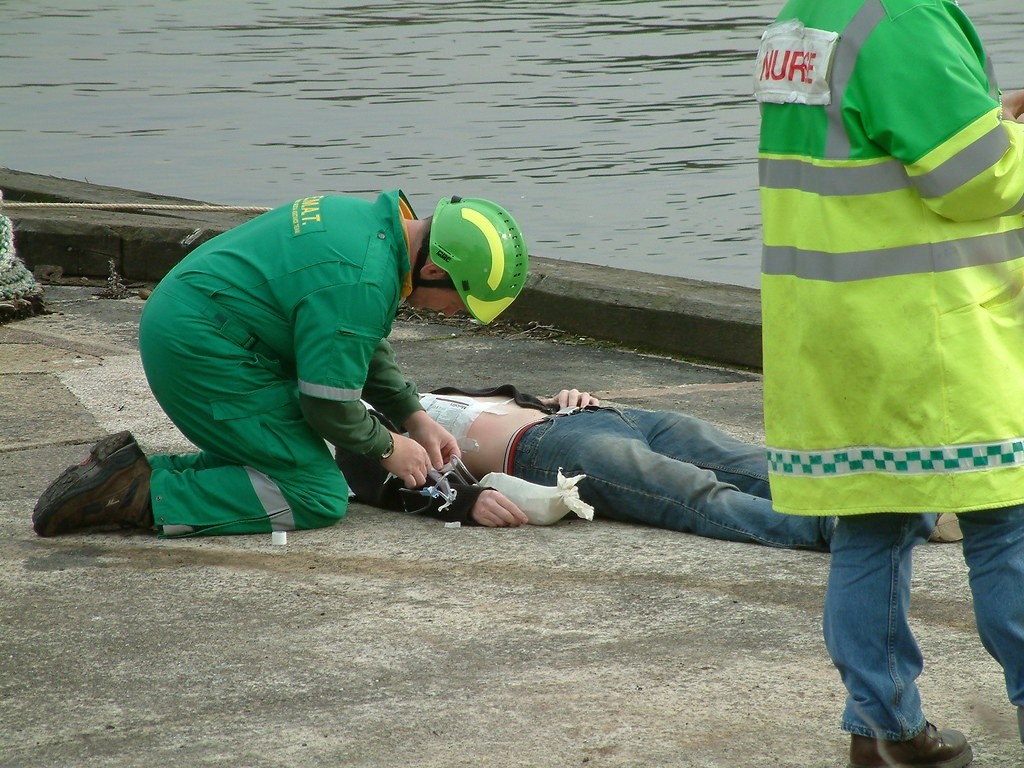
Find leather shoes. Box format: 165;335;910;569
846;721;973;768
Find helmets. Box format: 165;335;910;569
429;195;528;325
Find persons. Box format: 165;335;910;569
751;1;1023;766
34;188;530;537
335;385;963;552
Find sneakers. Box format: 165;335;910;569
33;441;153;537
32;430;136;523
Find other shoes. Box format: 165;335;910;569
931;512;963;543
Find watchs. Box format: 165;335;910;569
381;432;395;461
